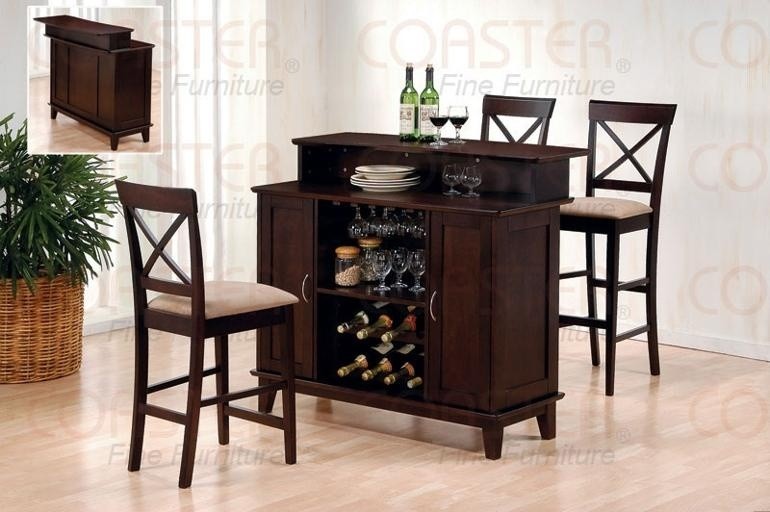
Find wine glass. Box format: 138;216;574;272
373;246;427;292
460;166;482;198
448;106;468;144
443;164;463;196
428;109;449;146
351;203;427;239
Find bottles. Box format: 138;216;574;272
358;240;383;282
420;64;439;143
400;62;419;142
337;301;425;390
335;246;362;286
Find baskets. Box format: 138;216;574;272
0;262;87;386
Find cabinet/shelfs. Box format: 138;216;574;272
250;132;590;462
34;16;155;151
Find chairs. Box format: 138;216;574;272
481;94;556;146
115;180;301;488
560;99;678;396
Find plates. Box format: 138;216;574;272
350;164;421;193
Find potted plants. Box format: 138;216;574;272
0;113;128;384
